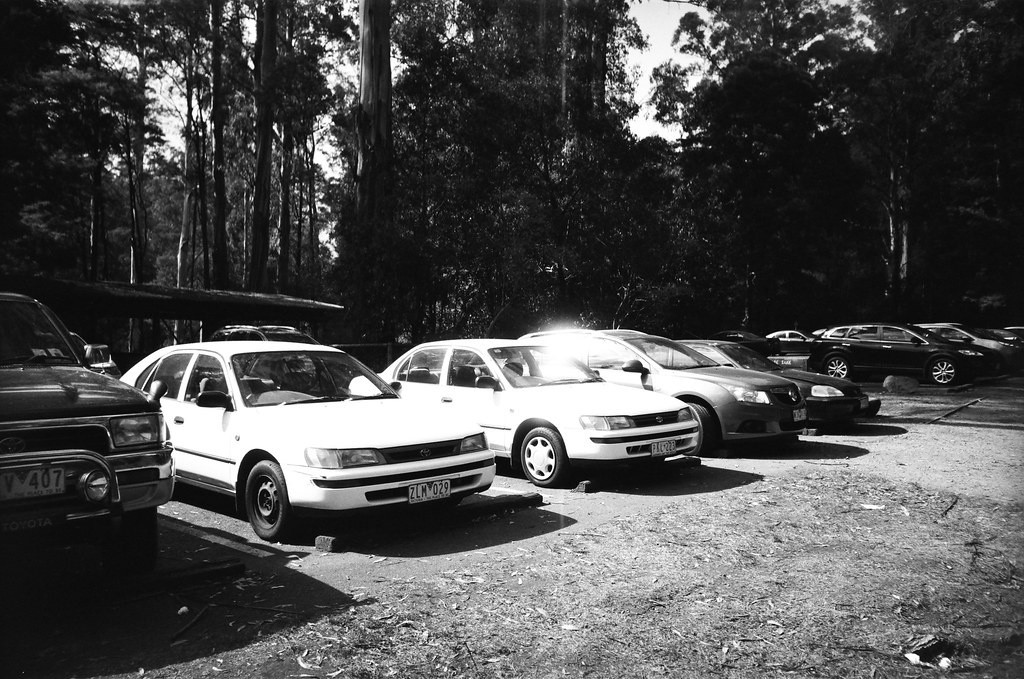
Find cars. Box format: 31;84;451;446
209;323;347;394
910;322;1024;371
703;331;781;358
349;338;701;487
765;330;816;354
113;339;497;540
805;321;999;387
672;337;885;438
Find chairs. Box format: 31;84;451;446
500;363;525;377
456;364;480;387
197;378;221;392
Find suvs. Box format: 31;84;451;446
514;328;811;456
0;292;176;575
